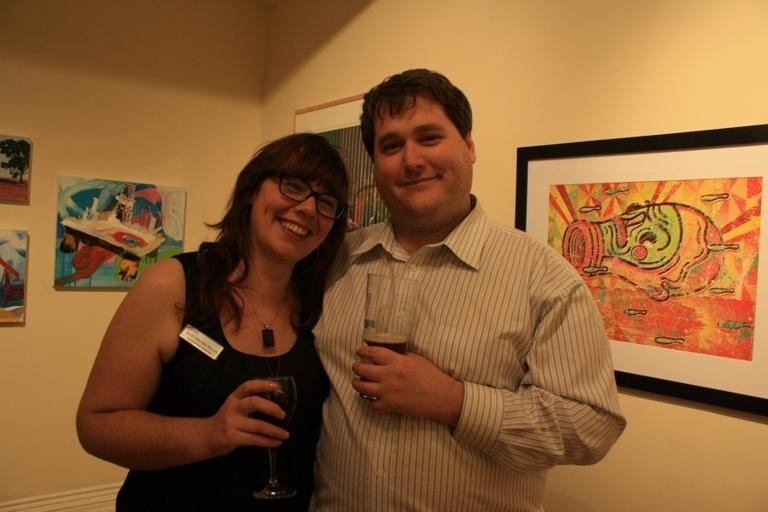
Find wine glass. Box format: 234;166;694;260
252;377;297;500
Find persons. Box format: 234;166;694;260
305;69;628;512
75;131;350;512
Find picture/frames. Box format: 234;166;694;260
292;92;390;230
515;123;768;420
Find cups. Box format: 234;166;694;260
360;274;420;399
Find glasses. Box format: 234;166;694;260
269;172;349;220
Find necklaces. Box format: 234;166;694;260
234;268;291;348
